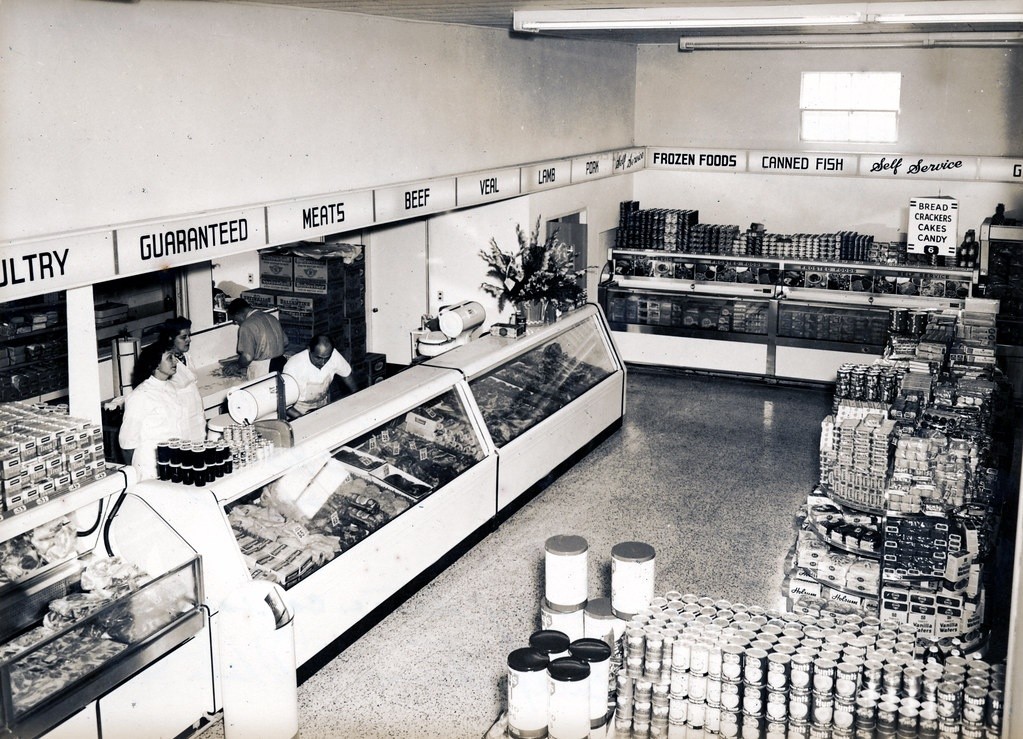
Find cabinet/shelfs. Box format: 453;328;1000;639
0;301;69;404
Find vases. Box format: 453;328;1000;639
519;299;543;326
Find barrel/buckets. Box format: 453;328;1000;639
507;534;657;739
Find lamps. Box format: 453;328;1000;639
511;0;1023;54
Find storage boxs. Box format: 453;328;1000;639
241;245;386;387
0;314;47;367
612;200;1000;639
0;401;107;511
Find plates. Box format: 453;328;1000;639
685;263;694;269
807;273;872;292
718;309;730;330
946;281;968;298
784;277;799;287
656;262;670;274
683;316;693;326
696;264;715;282
876;276;945;296
702;318;711;328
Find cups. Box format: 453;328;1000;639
157;425;274;486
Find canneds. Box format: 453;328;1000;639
506;535;1007;739
216;424;273;471
835;309;928;403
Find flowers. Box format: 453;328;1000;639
479;214;600;314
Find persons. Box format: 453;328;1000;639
118;339;190;483
159;315;206;443
280;335;359;420
227;297;290;382
